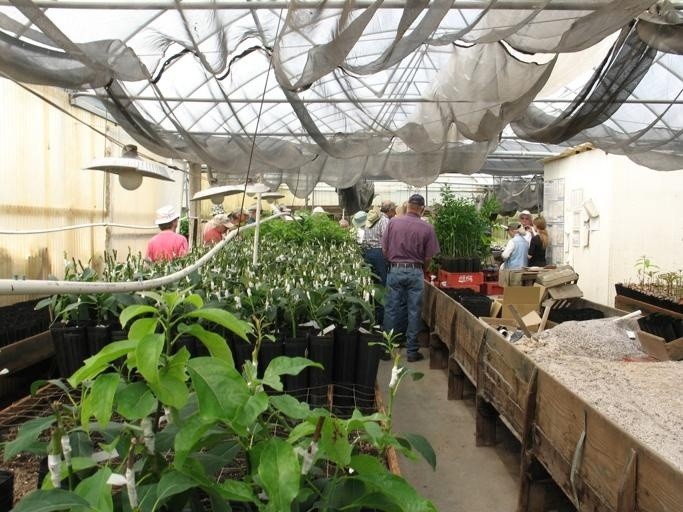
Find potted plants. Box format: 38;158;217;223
613;252;682;343
35;205;384;411
431;188;496;273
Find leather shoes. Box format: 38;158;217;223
407;353;423;362
381;353;391;360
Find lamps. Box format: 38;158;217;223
259;192;283;204
189;177;245;206
241;183;269;197
79;142;179;199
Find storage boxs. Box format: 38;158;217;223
489;283;545;320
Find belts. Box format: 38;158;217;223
391;263;421;267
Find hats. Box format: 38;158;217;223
154;205;180;225
381;200;398;211
408;194;424;206
518;210;533;221
209;201;266;229
365;206;380;228
351;210;367;229
505;222;520;231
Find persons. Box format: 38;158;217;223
203;208;248;244
499;221;530;278
526;217;549;268
143;204;187;260
517;209;538;249
311;193;442;362
